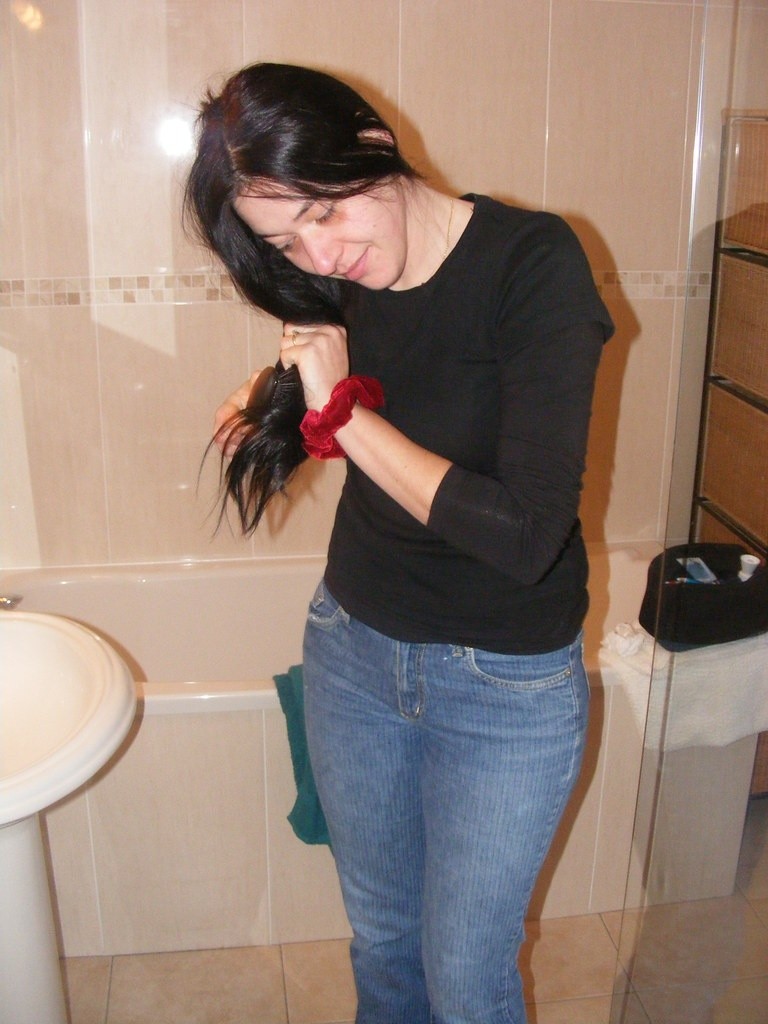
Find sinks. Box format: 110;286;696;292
1;608;138;828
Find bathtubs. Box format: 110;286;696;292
2;532;765;962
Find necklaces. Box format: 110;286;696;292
419;195;454;284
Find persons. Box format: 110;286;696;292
180;62;615;1024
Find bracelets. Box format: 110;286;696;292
299;375;387;461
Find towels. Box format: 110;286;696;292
271;662;335;863
597;615;768;756
